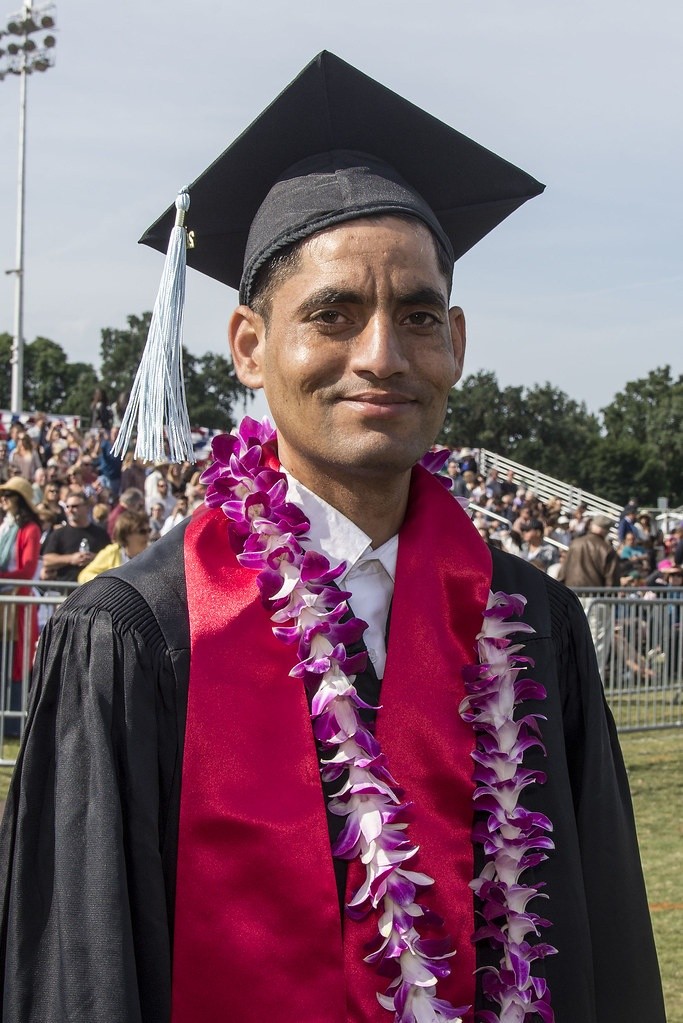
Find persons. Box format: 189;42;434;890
78;510;152;584
0;420;207;556
0;50;667;1023
42;492;111;595
430;445;683;678
90;388;113;429
556;516;621;687
0;477;44;681
106;391;130;441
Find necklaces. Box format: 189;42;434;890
199;415;559;1023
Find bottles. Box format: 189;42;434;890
79;538;90;566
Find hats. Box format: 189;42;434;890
519;519;543;530
0;476;38;514
635;510;652;519
109;50;548;464
593;515;613;529
460;465;475;475
621;505;638;514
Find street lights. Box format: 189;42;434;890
1;1;57;415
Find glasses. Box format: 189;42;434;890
521;528;531;532
127;528;152;535
66;504;79;508
